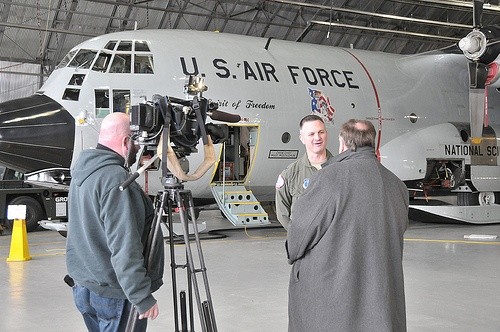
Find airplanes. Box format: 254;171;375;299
0;1;500;226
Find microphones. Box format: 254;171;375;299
207;110;242;123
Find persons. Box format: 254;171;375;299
284;118;410;331
275;115;335;232
64;112;165;332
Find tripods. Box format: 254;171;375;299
123;177;218;332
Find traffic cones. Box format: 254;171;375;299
5;219;32;262
